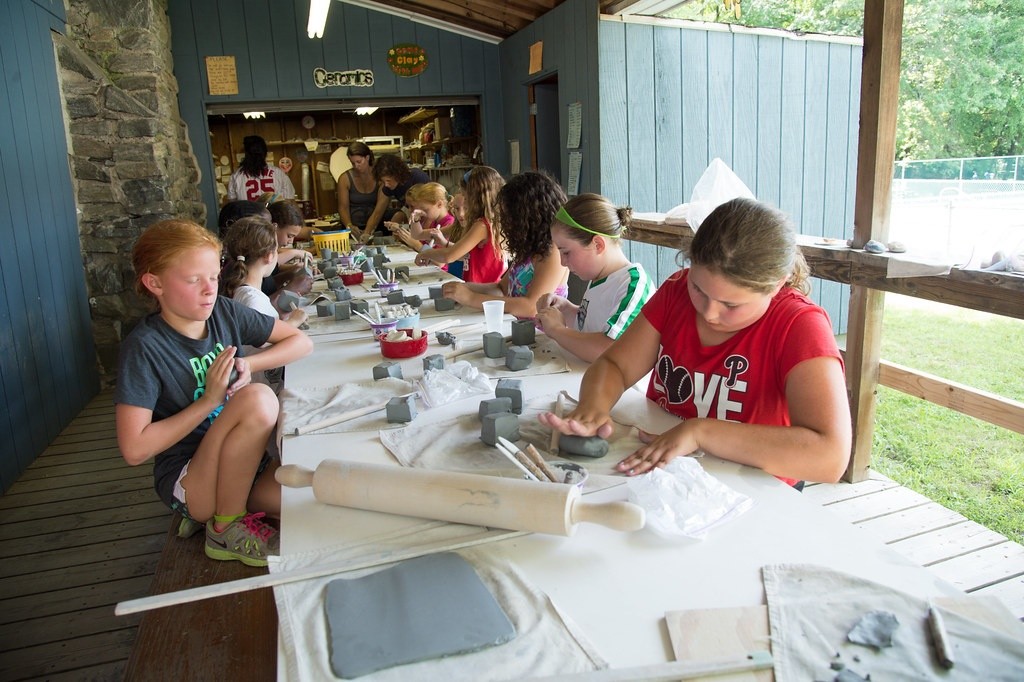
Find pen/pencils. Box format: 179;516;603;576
363;309;376;322
371;269;382;284
387;269;390;283
371;289;380;292
353;310;374;323
359;259;366;267
360;283;370;293
352;257;359;270
358;246;363;251
375;303;381;324
495;443;540;481
351;233;358;242
377;270;387;284
392;268;395;283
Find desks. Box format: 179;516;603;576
274;240;1024;682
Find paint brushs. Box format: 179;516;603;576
497;436;551;481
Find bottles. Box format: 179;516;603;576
441;143;447;167
434;149;442;167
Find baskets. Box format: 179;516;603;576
311;228;350;257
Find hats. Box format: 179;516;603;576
244;136;267;156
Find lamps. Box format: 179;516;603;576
352;106;380;115
305;0;331;40
243;112;265;119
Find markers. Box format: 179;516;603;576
928;608;955;668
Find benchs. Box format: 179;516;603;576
126;505;278;682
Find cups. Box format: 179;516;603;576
482;300;506;333
423;151;434;168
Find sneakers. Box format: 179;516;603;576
174;518;201;538
205;512;279;566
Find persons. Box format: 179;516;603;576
338;141;397;246
216;199;322;396
535;191;659;395
361;154;433;242
225;134;295;203
536;197;852;493
113;218;313;565
440;167;570;335
383;164;509;284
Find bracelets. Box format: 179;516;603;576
414;220;419;223
445;240;449;248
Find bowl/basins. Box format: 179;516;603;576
354;256;367;265
370;318;399;341
352;244;365;251
377;329;428;359
522;461;588;492
340;257;355;267
377;280;399;298
338;272;363;286
380;312;421;330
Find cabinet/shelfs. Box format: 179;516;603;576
397;109;478;171
